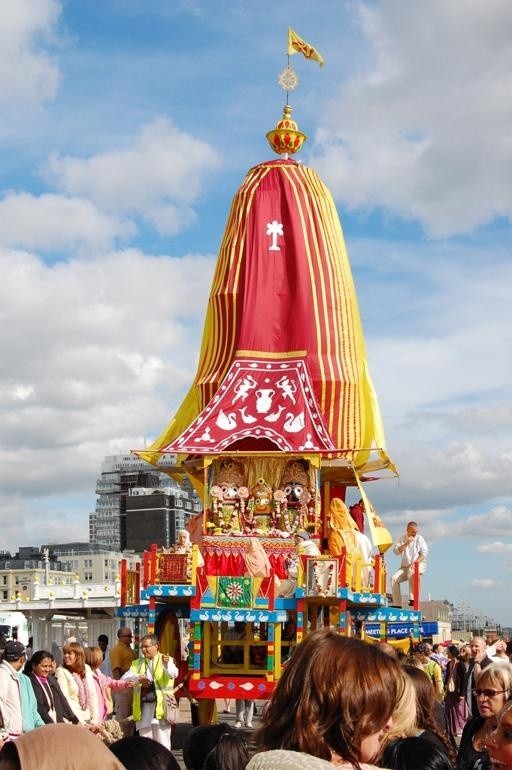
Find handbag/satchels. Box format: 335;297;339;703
162;694;181;728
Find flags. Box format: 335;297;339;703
287;26;325;69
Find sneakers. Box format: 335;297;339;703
233;722;253;730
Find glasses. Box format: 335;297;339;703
474;686;508;698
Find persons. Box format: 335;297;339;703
1;498;511;770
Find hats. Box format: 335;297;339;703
0;641;31;659
